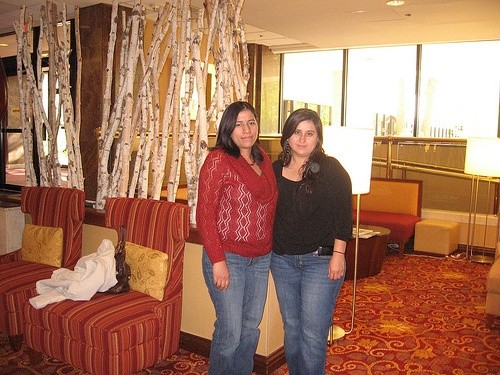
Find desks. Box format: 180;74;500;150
346;225;390;279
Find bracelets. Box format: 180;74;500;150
334;249;345;255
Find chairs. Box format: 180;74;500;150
1;186;190;375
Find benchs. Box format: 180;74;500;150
352;177;422;259
420;208;497;255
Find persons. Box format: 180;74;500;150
270;107;354;374
195;100;280;375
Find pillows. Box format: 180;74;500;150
118;240;169;301
20;224;64;269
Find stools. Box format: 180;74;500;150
413;219;459;256
